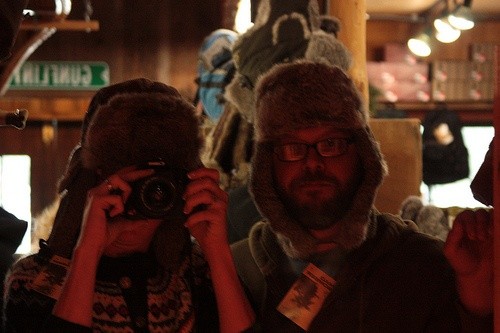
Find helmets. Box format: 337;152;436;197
197;28;241;73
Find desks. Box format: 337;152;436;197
369;118;424;215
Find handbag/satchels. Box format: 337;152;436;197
422;110;469;186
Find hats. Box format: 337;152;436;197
46;78;207;269
247;61;387;260
223;0;351;122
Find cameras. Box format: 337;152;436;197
105;157;203;222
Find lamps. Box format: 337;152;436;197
407;0;477;58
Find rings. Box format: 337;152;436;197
103;178;113;192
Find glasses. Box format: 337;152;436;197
270;137;355;163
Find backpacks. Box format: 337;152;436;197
194;61;236;121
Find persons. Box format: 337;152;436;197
0;78;263;333
200;62;493;333
